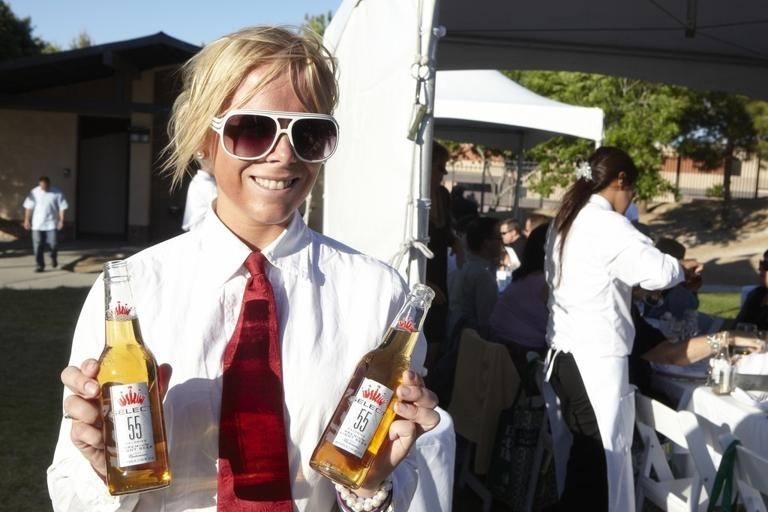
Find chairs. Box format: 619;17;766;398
536;359;638;500
628;385;722;512
456;329;546;511
704;412;767;511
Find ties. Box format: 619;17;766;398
218;252;301;510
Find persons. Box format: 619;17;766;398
630;238;768;447
23;176;69;275
177;161;221;233
427;141;556;445
543;146;704;512
45;28;454;510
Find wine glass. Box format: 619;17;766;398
642;289;665;308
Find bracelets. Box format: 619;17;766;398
324;475;393;512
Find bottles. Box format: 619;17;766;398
709;330;734;396
94;258;174;497
308;283;436;490
687;271;703;290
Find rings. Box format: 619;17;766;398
62;404;72;421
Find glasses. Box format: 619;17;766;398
211;108;339;163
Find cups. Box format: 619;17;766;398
736;321;757;337
